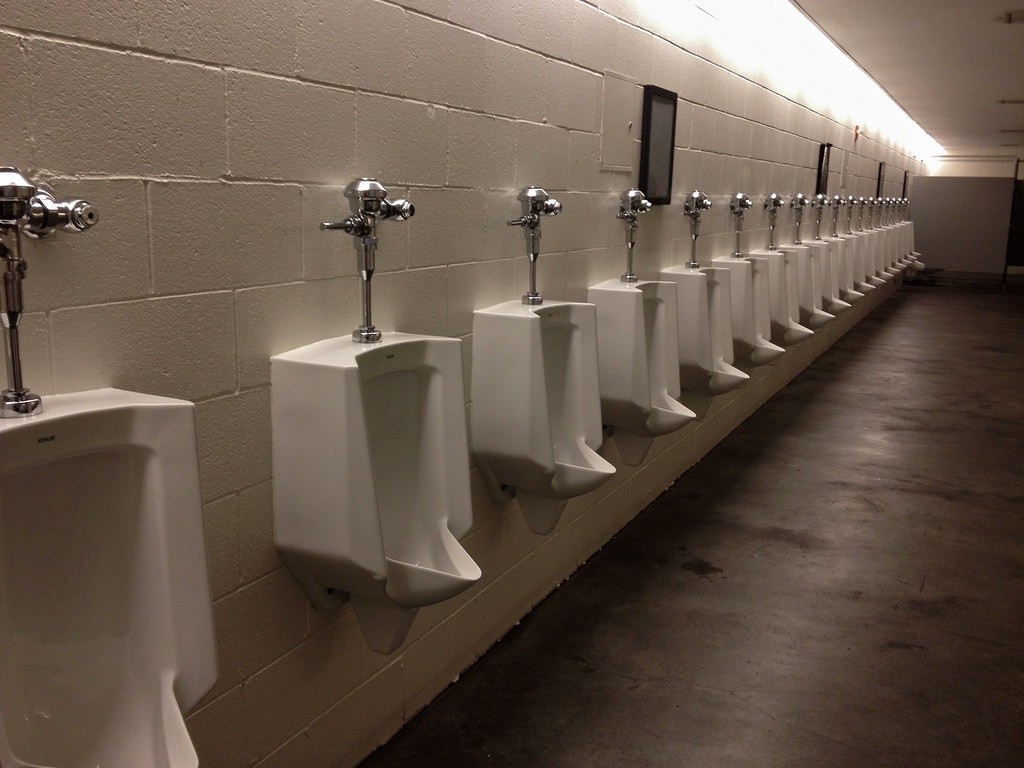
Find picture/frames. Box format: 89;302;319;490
876;161;885;201
815;143;831;205
902;171;909;200
637;82;679;208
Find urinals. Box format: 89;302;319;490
0;168;924;763
